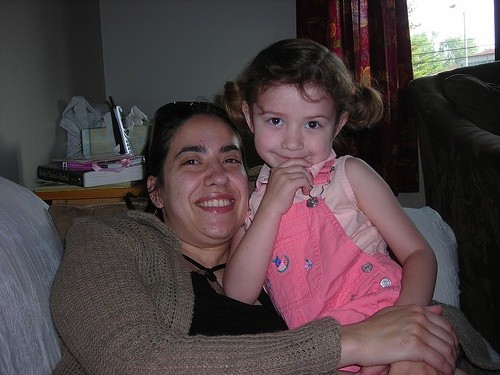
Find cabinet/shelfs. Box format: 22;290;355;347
32;179;149;207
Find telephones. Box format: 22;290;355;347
108;95;135;155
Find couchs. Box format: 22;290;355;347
408;59;500;337
0;173;459;375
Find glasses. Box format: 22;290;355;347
148;101;231;166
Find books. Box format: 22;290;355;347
37;155;143;187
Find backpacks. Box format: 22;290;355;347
443;73;500;135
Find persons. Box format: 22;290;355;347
49;101;500;375
222;38;467;375
